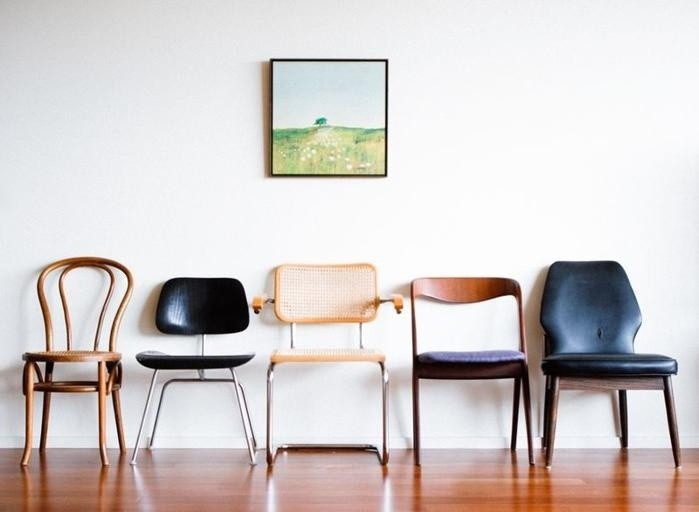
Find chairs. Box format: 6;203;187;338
539;261;681;468
133;278;257;469
20;258;129;468
253;264;404;468
412;277;537;470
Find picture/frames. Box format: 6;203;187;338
267;56;391;179
